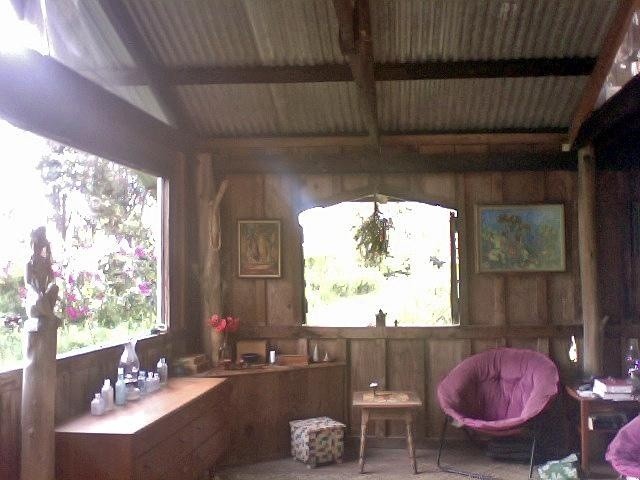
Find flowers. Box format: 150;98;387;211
210;313;240;333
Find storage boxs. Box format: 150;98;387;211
289;416;347;468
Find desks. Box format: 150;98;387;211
563;383;640;475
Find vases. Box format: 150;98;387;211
221;331;230;359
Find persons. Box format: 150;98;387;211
242;230;278;272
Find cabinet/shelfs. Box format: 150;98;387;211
55;378;229;480
190;357;347;469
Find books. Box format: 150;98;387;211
594;377;633;395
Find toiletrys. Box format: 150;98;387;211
90;357;170;416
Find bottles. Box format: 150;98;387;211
90;379;114;417
115;338;169;405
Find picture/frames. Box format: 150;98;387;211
236;218;282;278
473;201;567;274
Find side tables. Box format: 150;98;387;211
351;390;423;474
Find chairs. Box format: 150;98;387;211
436;348;559;479
603;415;640;480
